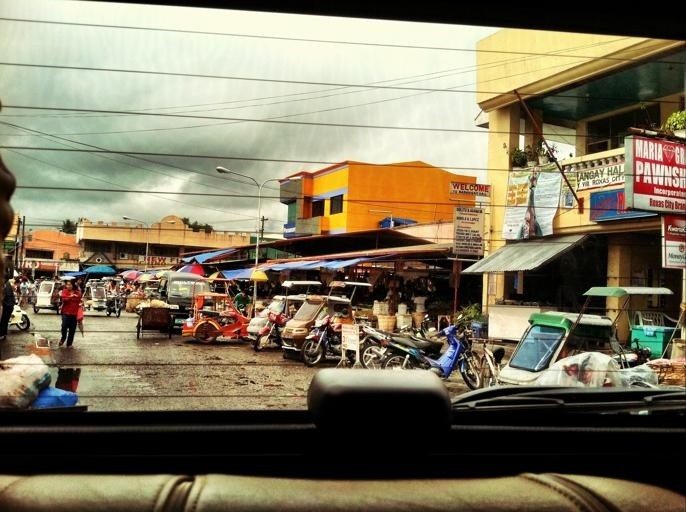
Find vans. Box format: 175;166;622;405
157;271;214;334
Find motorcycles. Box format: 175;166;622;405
106;294;122;318
245;293;481;390
7;302;30;332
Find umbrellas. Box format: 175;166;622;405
232;270;279;302
117;270;158;286
84;264;116;273
176;261;206;277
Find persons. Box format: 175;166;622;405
105;280;121;297
412;287;431;314
230;289;251;339
0;275;86;349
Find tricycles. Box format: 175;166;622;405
33;280;65;315
181;277;255;344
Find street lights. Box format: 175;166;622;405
215;165;304;315
121;216;176;274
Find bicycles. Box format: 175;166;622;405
469;336;505;387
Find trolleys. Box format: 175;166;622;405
134;300;180;340
